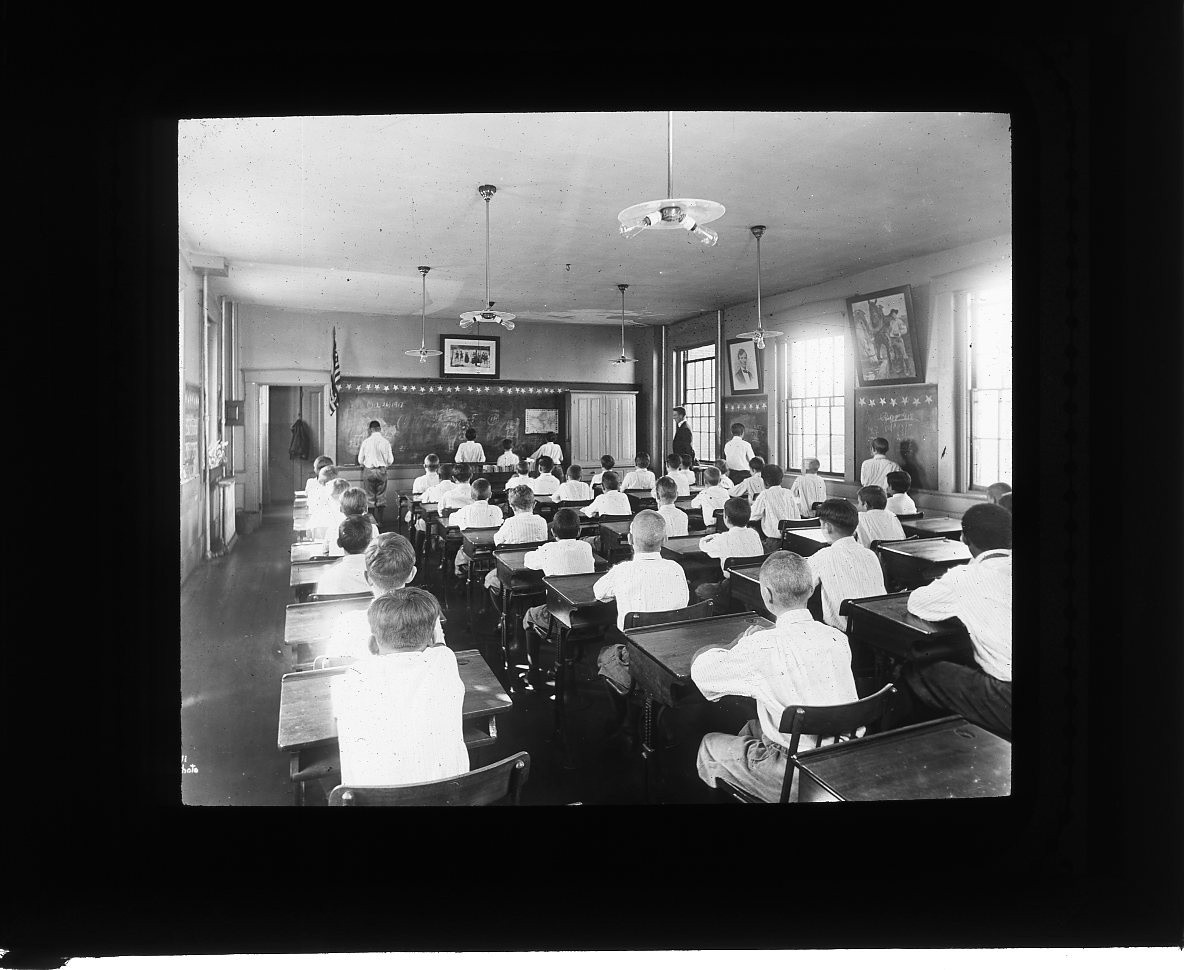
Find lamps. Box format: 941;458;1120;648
458;182;517;330
618;112;727;248
404;266;444;363
609;283;640;367
736;225;785;351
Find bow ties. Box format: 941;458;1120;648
742;368;751;374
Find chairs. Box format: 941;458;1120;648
602;597;713;748
715;682;897;806
329;750;532;808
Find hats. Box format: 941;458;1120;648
888;308;897;316
313;456;333;474
370;421;380;428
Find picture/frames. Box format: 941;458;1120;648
440;333;500;379
845;283;925;387
726;338;763;395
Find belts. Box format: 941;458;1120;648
371;466;386;469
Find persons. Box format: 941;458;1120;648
853;309;912;377
674;407;698;464
289;420;1013;805
455;351;489;365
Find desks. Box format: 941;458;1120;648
272;484;1010;804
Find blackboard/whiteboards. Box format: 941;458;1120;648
335;376;569;470
724;394;768;464
854;383;938;491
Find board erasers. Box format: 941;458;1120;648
344;464;355;466
